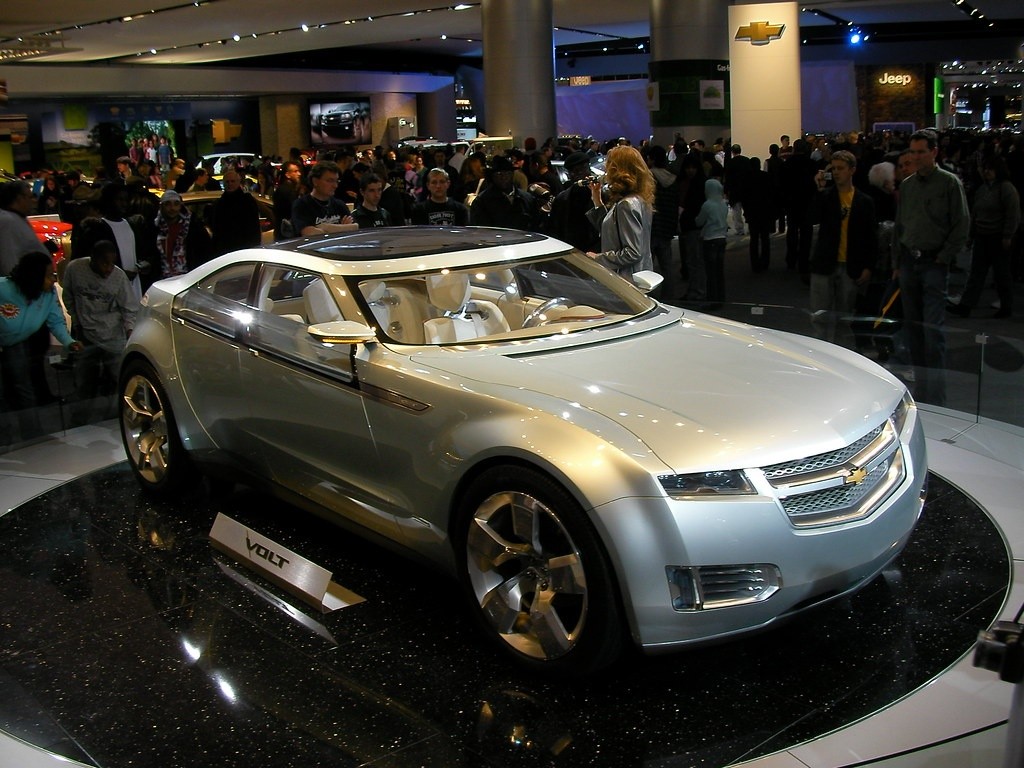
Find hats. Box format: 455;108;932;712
482;155;521;170
161;190;180;202
565;153;590;168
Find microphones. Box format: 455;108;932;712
578;176;607;187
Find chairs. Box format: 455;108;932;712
301;280;390;331
424;271;510;346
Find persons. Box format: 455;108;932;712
0;154;273;415
585;143;654;286
130;134;175;171
648;126;1024;407
272;137;649;252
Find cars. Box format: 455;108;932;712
195;152;283;177
322;103;369;135
116;224;926;686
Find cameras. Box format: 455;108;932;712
823;173;832;180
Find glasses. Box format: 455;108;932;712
496;171;512;176
566;169;585;179
